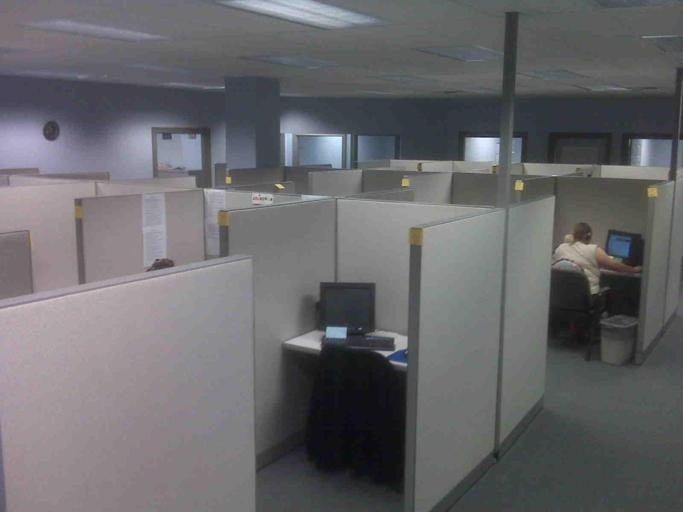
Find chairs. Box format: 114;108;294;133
318;342;406;483
550;264;614;362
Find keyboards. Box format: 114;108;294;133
321;334;395;351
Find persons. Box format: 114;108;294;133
551;222;642;312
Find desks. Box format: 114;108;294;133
551;256;641;282
277;319;410;383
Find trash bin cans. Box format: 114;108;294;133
599;314;638;367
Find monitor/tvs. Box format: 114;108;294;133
605;229;644;267
319;281;376;335
